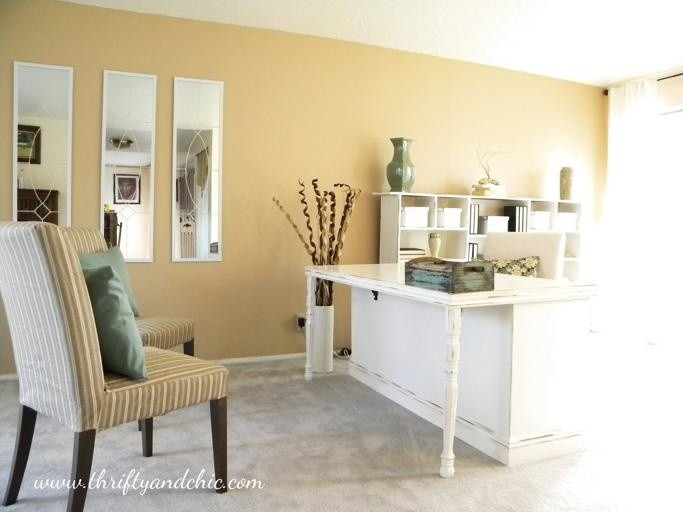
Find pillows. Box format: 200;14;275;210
84;266;149;380
470;255;539;278
78;246;139;315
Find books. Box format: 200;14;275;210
473;244;477;261
400;246;427;263
470;202;528;235
469;243;474;261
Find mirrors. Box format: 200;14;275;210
13;60;73;226
172;78;224;262
100;69;158;263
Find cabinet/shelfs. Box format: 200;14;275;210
373;192;583;271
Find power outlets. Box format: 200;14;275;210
297;313;305;331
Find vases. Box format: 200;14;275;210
560;167;574;200
386;136;416;193
313;305;334;373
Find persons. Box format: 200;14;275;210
117;179;136;200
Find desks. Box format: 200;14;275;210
304;263;602;478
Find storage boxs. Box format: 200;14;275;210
478;216;509;234
438;208;463;228
401;207;429;228
531;211;551;229
559;213;578;229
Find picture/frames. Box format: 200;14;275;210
114;174;140;204
18;125;42;164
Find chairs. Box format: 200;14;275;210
65;225;195;431
0;221;230;512
482;232;565;284
